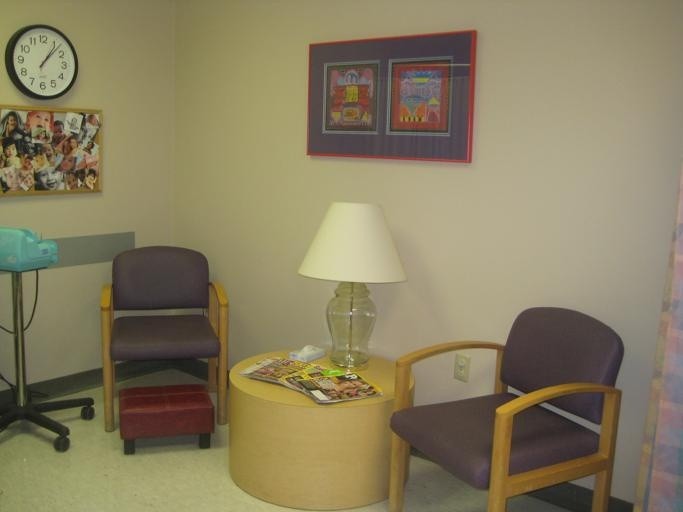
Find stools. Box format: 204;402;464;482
116;384;215;455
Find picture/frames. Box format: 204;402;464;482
307;29;477;163
0;104;103;196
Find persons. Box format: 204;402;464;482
0;110;101;193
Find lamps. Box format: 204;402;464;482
298;202;407;370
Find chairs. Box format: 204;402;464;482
98;246;229;433
390;307;625;512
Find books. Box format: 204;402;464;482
238;355;384;405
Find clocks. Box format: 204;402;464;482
6;25;78;100
